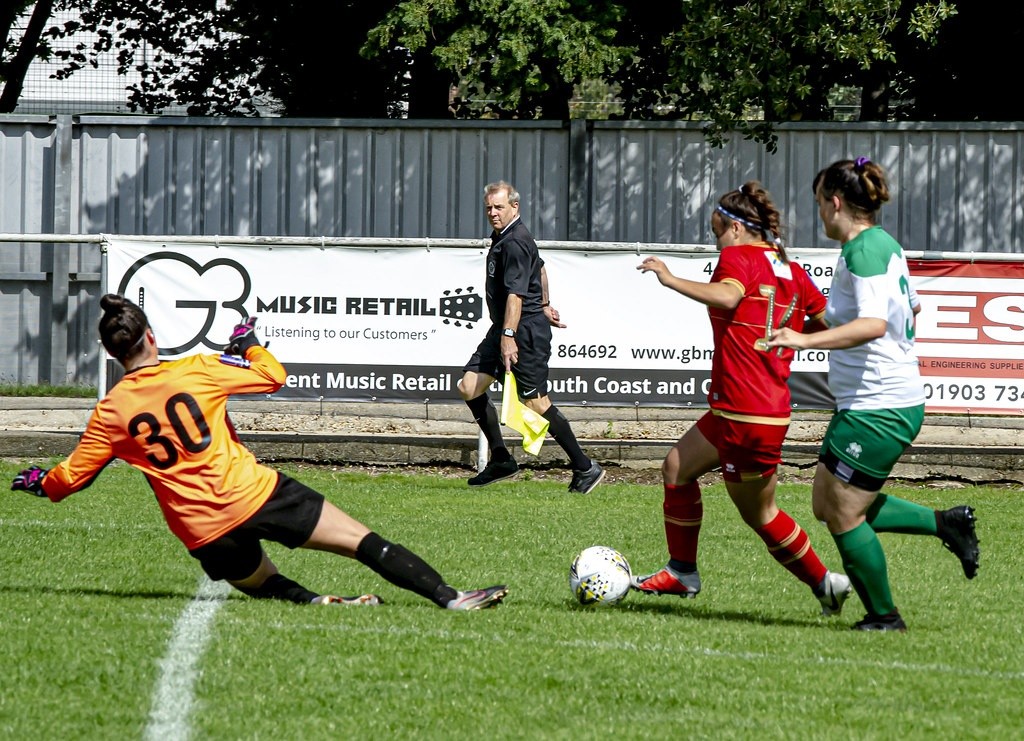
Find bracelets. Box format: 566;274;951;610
541;301;549;308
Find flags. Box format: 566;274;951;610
499;370;550;457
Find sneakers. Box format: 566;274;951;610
567;460;607;497
628;566;701;598
311;592;384;608
848;608;907;632
941;503;981;579
814;568;852;618
446;580;507;611
466;455;521;487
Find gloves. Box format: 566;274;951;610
224;315;262;362
10;465;51;498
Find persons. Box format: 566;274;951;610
9;293;508;610
459;180;606;494
628;179;854;614
764;157;979;630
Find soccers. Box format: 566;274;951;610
568;546;632;609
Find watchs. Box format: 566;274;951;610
500;329;516;337
